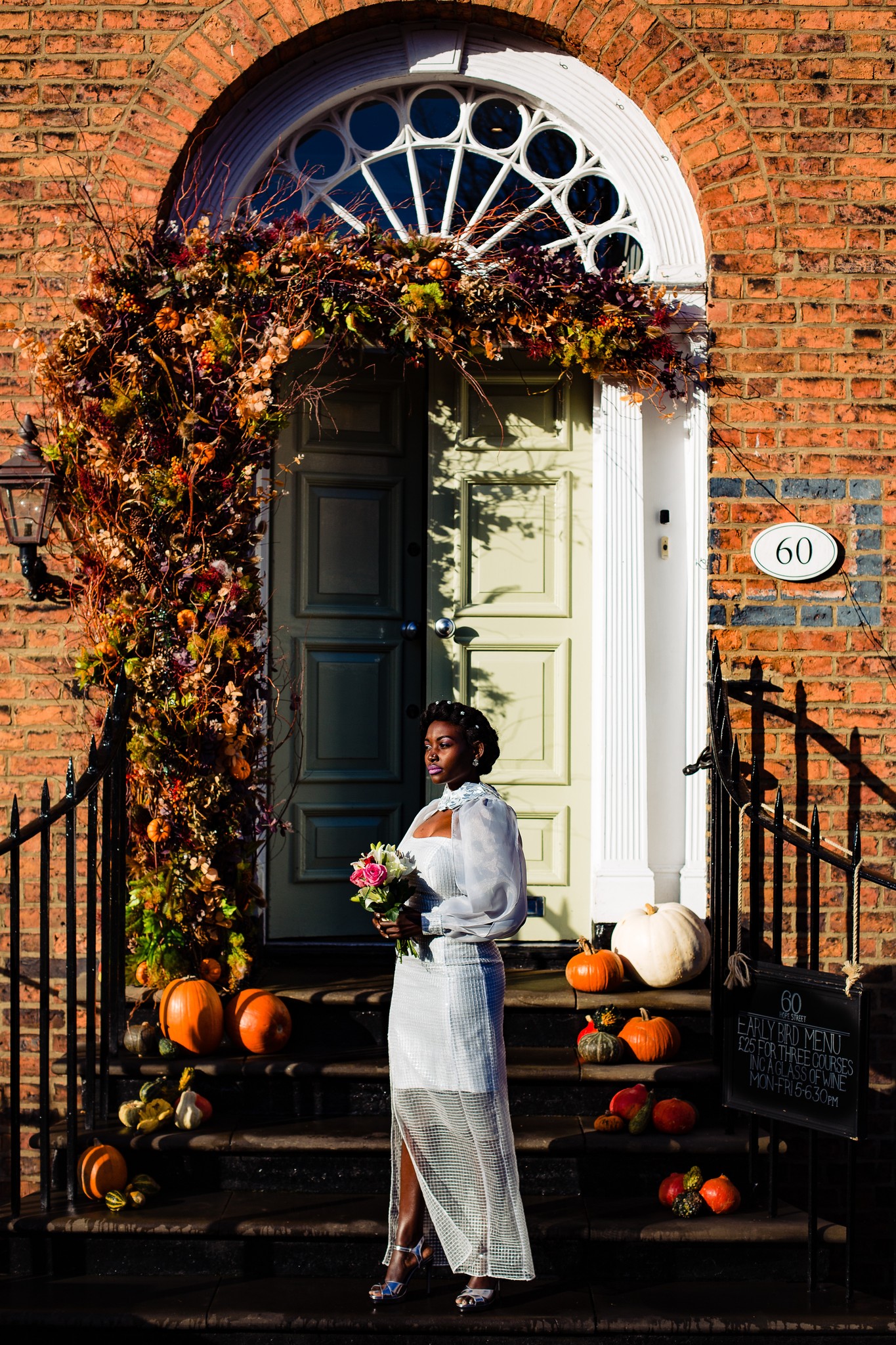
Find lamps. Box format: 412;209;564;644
1;412;72;593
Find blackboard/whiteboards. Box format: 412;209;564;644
722;958;861;1142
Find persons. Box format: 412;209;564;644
367;697;536;1314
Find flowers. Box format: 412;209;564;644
347;841;426;964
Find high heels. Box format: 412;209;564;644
369;1235;434;1302
456;1276;501;1313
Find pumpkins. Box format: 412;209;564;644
565;936;624;993
230;758;251;782
617;1007;681;1063
191;442;215;465
428;258;451;280
156;307;179;330
118;1067;212;1133
123;1021;157;1056
147;818;171;843
160;976;225;1059
227;988;291;1054
136;961;151;987
95;641;116;660
237;251;260;273
594;1083;696;1135
658;1166;741;1218
576;1003;627;1063
157;1037;179;1058
176;609;199;637
198;958;221;983
76;1138;128;1200
105;1174;161;1211
610;901;711;989
292;330;314;350
